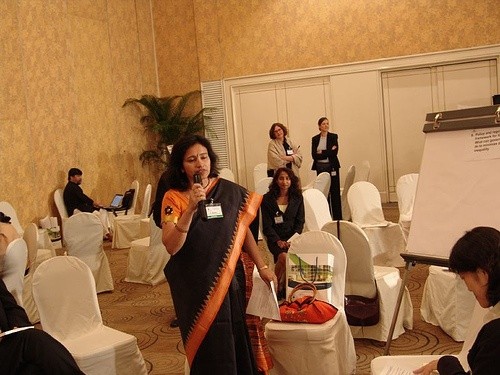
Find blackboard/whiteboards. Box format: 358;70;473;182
399;105;500;266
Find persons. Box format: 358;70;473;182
0;212;30;279
311;117;342;220
267;122;302;177
0;279;85;375
147;134;278;375
261;167;305;300
63;167;114;242
413;226;500;375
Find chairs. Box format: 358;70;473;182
0;162;500;374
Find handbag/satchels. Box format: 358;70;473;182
288;253;335;305
272;283;339;325
344;281;379;326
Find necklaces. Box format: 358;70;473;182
203;177;210;189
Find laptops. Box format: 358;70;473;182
102;194;124;209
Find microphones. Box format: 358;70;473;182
193;174;207;222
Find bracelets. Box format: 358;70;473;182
258;264;270;270
175;224;188;233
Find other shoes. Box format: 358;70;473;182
276;291;286;301
